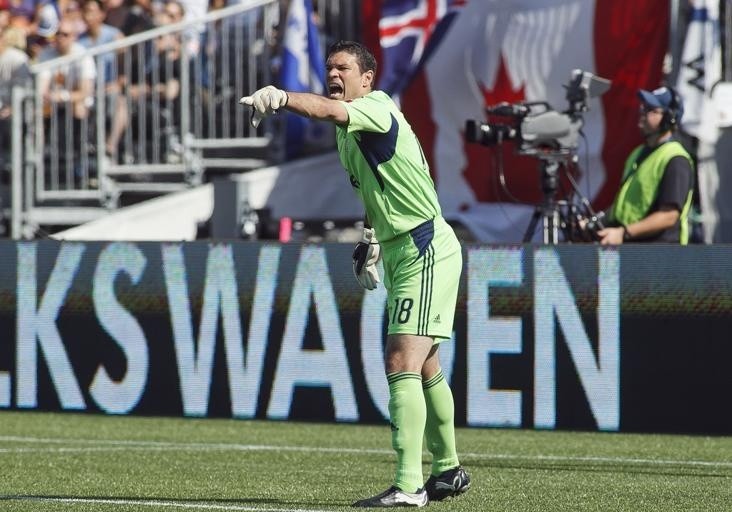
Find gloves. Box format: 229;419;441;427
237;86;288;127
352;228;384;290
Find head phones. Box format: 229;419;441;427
660;84;680;130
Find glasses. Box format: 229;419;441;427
154;6;184;47
639;100;660;112
53;30;72;36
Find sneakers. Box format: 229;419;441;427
355;485;432;507
426;466;471;500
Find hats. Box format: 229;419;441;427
32;5;56;36
637;84;685;103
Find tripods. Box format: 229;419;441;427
522;158;601;245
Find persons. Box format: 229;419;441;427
237;38;471;508
